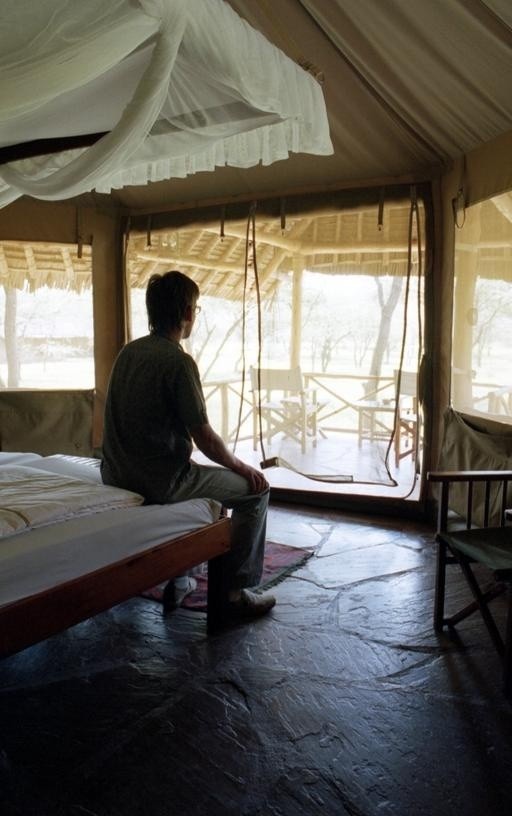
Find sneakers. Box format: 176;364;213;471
162;573;198;614
222;587;276;630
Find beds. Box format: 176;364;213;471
0;448;233;662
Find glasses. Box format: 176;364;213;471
192;303;202;316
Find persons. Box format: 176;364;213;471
96;271;279;629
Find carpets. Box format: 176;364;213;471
135;540;315;612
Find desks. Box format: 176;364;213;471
281;396;330;440
353;401;410;450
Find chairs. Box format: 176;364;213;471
487;385;512;415
393;369;427;468
248;366;320;455
425;471;512;665
